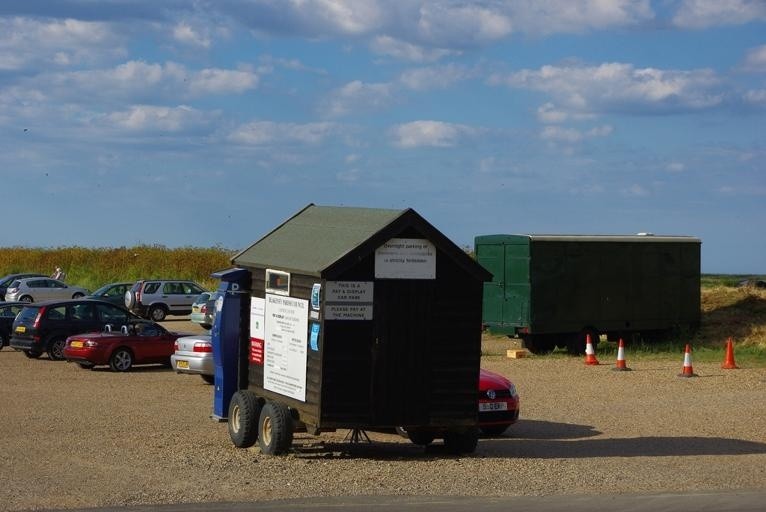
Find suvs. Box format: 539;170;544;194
734;279;766;289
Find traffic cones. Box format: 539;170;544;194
676;344;700;379
583;334;600;365
610;337;633;372
720;338;740;370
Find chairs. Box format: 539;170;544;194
104;324;111;332
121;325;128;334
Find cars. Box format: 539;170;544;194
392;363;524;443
0;271;219;374
168;329;215;385
